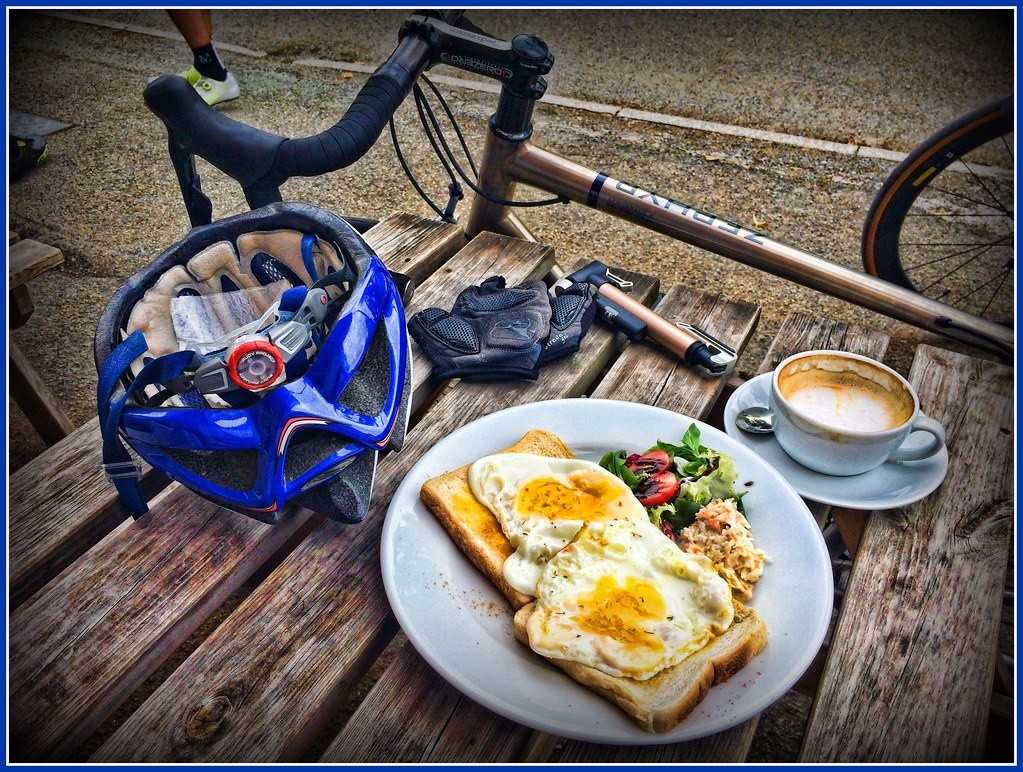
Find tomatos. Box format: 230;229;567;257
630;450;670;474
639;470;679;505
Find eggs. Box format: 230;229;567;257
525;518;734;680
469;454;651;594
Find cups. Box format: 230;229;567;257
769;349;945;478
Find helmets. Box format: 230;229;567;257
92;199;412;527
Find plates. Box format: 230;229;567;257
723;370;950;510
380;399;835;745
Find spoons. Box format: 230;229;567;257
734;406;772;436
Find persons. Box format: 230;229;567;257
147;9;240;106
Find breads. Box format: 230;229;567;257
422;431;766;732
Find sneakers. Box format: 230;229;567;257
9;133;49;185
146;63;240;107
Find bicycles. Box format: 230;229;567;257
138;10;1017;370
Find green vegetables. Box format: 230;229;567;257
600;422;747;529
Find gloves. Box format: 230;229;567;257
406;274;601;383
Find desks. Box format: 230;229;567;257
8;214;1017;765
